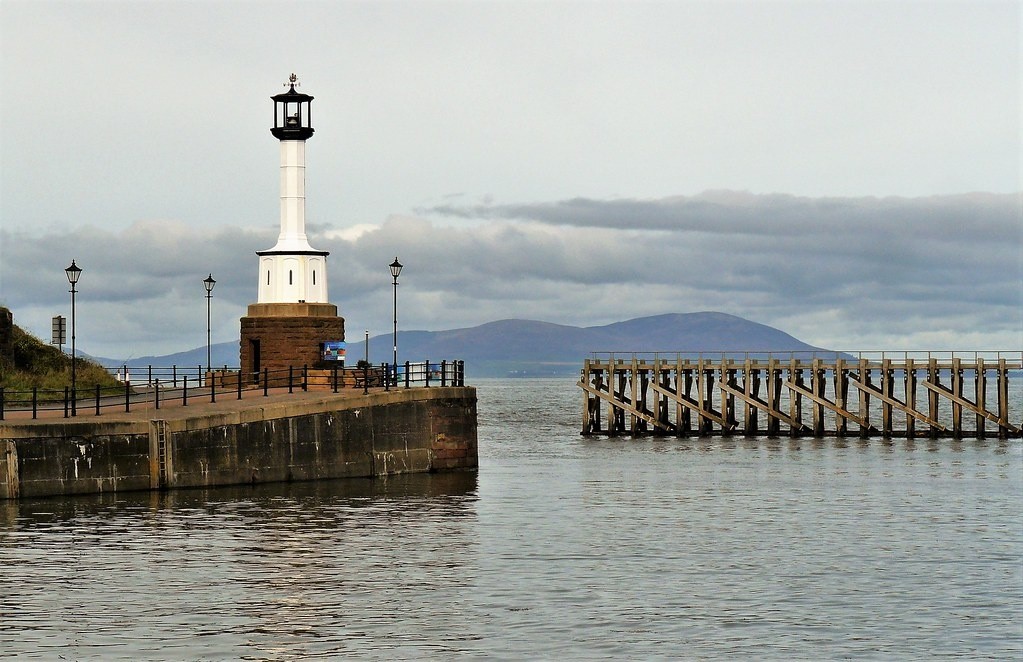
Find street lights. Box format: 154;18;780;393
65;260;83;416
202;274;217;372
389;257;403;386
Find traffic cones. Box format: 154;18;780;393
123;369;130;385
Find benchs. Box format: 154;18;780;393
351;369;398;388
